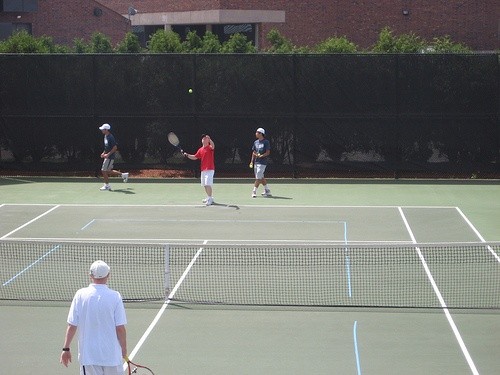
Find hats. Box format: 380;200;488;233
99;124;110;129
257;127;265;137
90;260;110;279
201;134;210;139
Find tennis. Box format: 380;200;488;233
189;88;193;93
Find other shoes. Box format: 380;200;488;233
100;186;111;191
123;173;128;182
202;199;214;202
261;190;271;196
206;199;213;205
252;193;256;197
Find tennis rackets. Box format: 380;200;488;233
167;131;187;155
124;355;154;375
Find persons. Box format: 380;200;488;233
61;260;127;375
99;124;128;190
182;135;215;205
250;128;271;197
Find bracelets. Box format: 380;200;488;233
62;348;69;351
